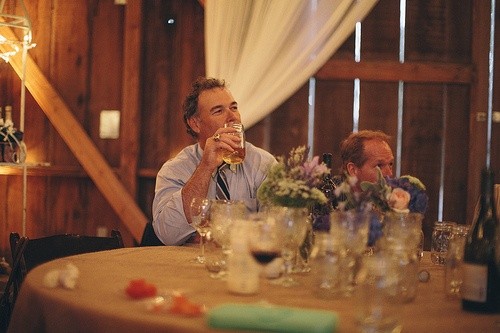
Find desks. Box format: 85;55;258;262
7;246;500;333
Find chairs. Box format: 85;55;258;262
8;229;124;302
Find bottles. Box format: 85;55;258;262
3;106;16;163
314;152;338;231
0;105;4;162
460;168;500;315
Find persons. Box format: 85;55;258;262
308;129;395;219
152;78;281;246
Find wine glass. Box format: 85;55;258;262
248;215;281;309
191;198;308;287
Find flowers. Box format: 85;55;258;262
357;165;430;218
260;144;329;210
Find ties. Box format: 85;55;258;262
215;161;231;201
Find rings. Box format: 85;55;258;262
213;133;220;142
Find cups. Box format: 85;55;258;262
445;225;470;297
221;122;246;165
430;221;458;265
311;211;423;333
226;221;260;295
